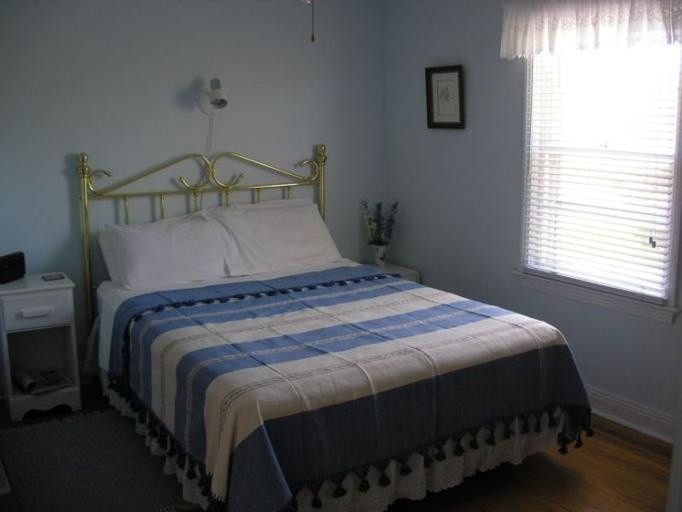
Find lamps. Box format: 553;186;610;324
197;76;229;118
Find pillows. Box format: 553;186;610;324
93;206;227;290
213;197;342;278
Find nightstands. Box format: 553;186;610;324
0;272;83;421
359;261;421;290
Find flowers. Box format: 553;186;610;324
361;199;399;244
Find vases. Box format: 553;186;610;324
365;242;389;268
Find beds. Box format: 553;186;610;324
77;144;596;507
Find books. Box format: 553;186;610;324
13;364;72;397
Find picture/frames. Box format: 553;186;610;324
424;64;467;129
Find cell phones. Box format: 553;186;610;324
42;274;64;281
